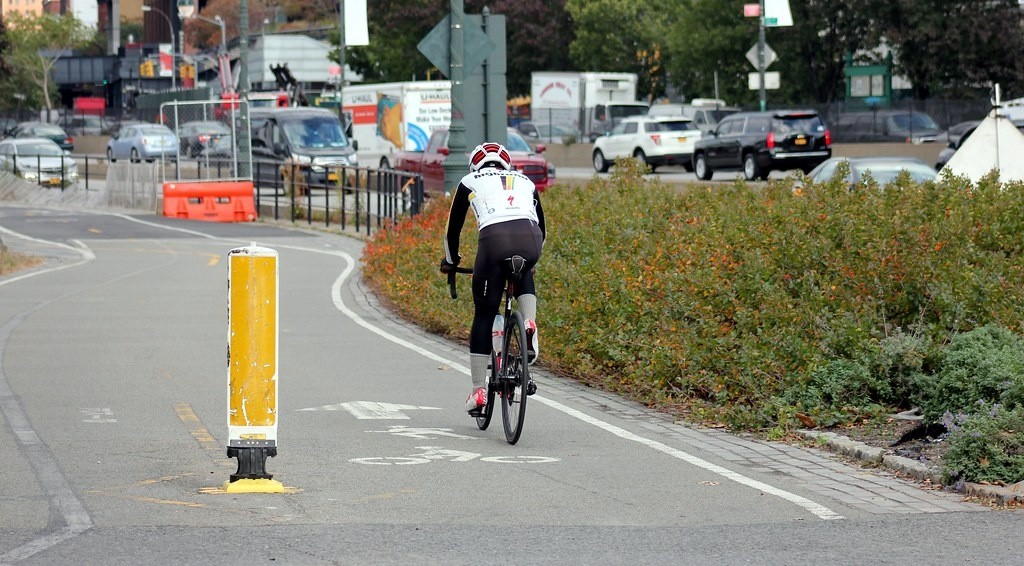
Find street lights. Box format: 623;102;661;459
140;5;176;90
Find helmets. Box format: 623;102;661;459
468;143;513;172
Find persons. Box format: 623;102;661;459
444;143;546;413
308;127;332;147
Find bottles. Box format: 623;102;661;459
491;311;504;355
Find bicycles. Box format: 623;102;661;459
440;254;538;445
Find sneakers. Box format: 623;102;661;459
518;319;539;366
465;388;488;412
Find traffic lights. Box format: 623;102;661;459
102;73;109;86
180;65;187;79
145;61;154;78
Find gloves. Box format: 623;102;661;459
440;258;460;270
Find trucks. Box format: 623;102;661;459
532;70;650;143
318;80;454;194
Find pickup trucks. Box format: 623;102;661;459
393;126;557;204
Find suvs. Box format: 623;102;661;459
592;114;704;174
692;108;831;182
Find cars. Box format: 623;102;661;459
176;119;233;159
106;123;179;165
0;138;78;189
58;113;157;139
512;121;591;146
935;85;1024;174
5;121;74;153
791;153;940;192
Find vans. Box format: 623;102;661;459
230;106;360;191
828;110;945;146
646;97;746;137
